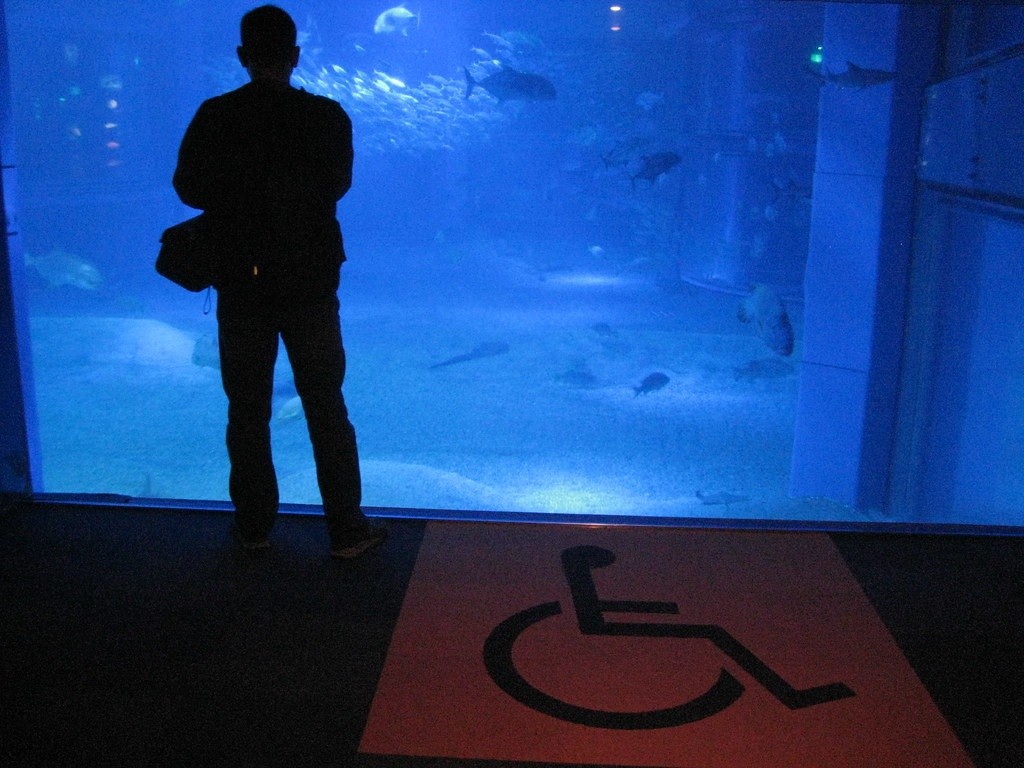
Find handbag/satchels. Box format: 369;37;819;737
156;213;214;293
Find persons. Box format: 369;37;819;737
172;5;390;557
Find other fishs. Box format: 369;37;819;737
1;0;907;508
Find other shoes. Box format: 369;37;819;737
331;516;390;560
231;513;270;549
